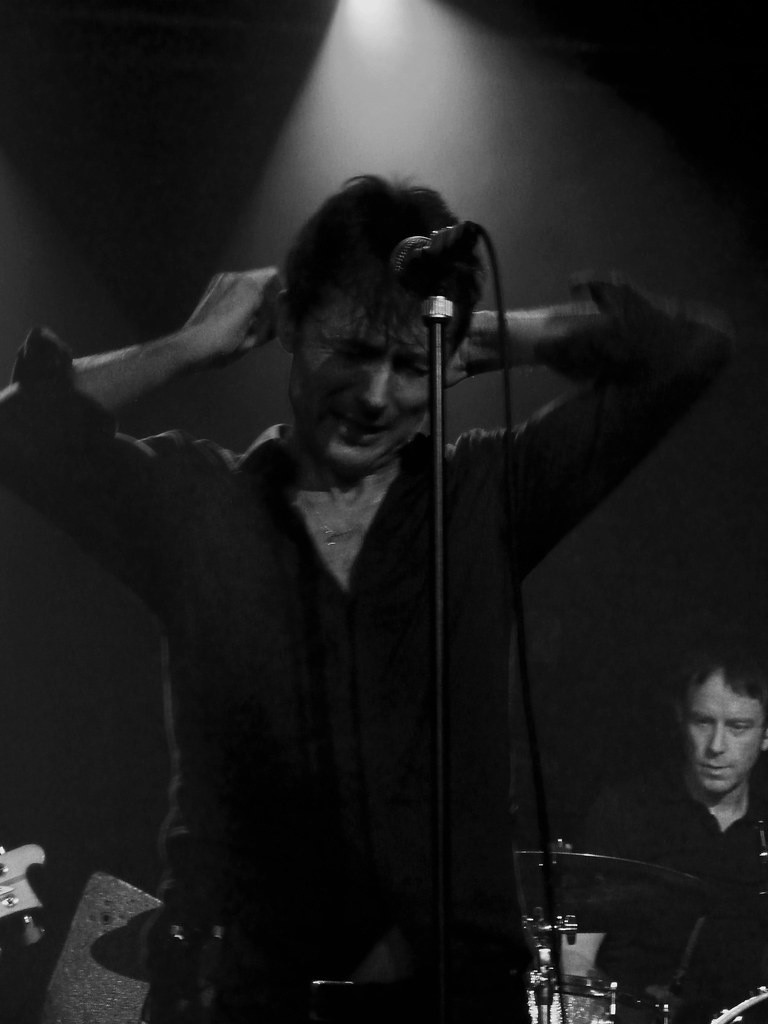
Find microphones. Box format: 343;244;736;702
392;220;479;299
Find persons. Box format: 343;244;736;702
0;173;738;1024
559;640;768;1024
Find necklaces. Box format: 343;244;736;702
300;476;391;549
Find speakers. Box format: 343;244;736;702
43;871;164;1024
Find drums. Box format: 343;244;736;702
520;912;611;978
672;984;768;1024
523;971;673;1024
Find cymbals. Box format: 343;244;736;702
513;849;701;887
